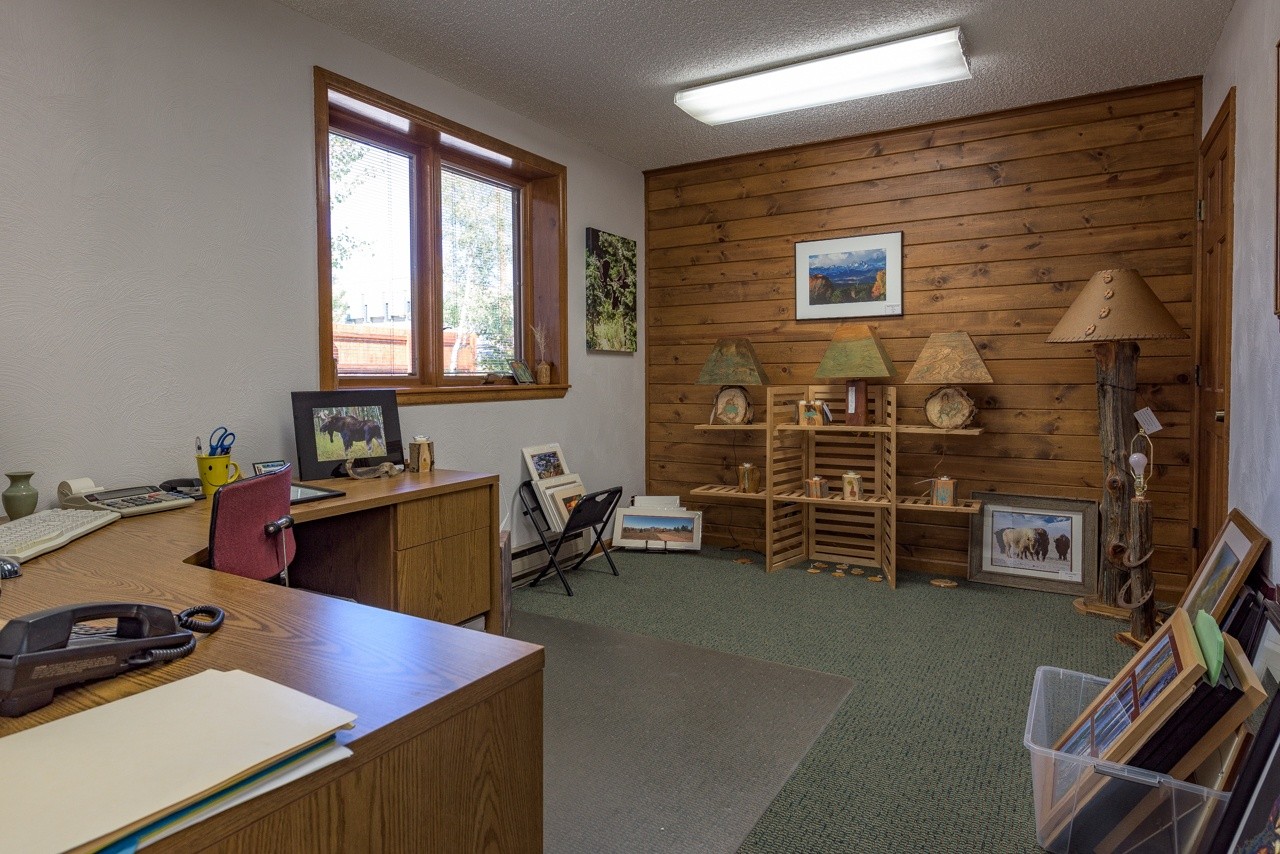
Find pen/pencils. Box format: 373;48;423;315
218;447;231;456
194;436;202;456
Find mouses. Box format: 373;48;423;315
1;559;25;580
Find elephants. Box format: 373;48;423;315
1002;527;1040;561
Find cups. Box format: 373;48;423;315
195;453;240;495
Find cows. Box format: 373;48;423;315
1054;534;1070;561
320;412;387;458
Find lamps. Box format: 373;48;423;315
1044;269;1191;626
674;26;972;126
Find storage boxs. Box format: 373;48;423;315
1023;664;1232;854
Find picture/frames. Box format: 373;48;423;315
290;390;406;482
611;507;702;551
506;359;536;385
521;443;587;532
1038;508;1280;854
966;491;1099;598
795;230;904;321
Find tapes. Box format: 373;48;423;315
57;477;106;503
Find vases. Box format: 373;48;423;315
536;361;550;385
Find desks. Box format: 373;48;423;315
0;469;544;854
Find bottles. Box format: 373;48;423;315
2;471;39;521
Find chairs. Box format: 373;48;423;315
519;480;622;597
209;463;296;588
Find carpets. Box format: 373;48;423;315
507;610;855;854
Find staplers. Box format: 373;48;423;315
159;478;207;500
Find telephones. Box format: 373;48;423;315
0;601;195;718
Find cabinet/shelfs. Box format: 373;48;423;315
689;384;985;589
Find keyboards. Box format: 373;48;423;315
0;507;122;562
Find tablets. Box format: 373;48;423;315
289;474;346;504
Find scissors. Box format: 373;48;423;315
208;426;235;456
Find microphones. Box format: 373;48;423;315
265;513;295;536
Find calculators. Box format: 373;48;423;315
63;483;196;517
